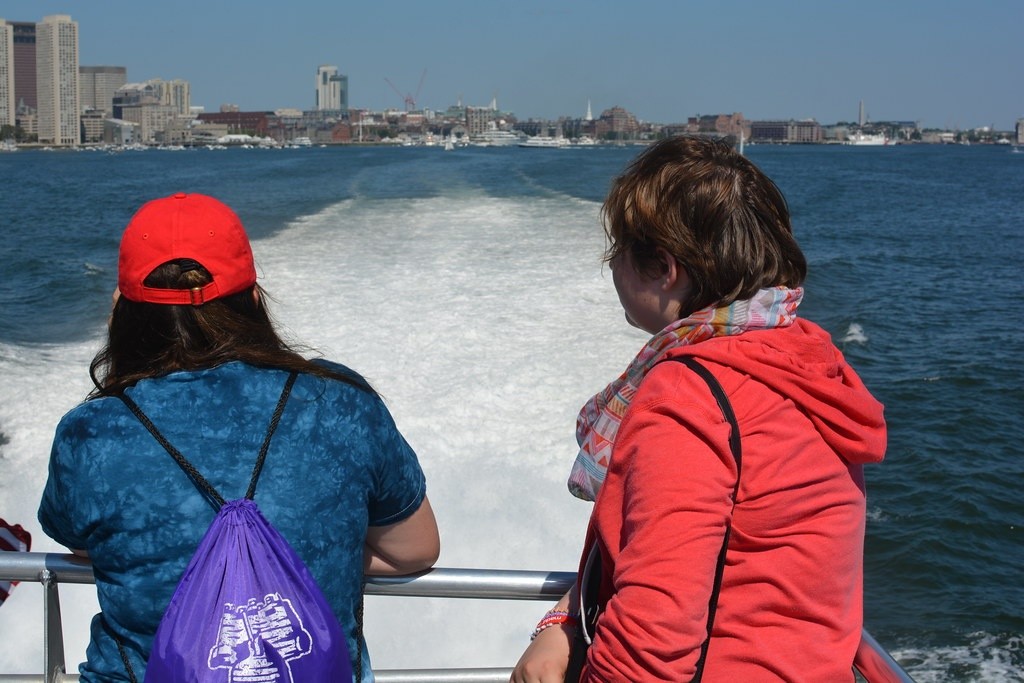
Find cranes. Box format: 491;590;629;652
383;66;429;110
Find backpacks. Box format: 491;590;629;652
108;366;356;683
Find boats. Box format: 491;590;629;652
156;142;186;152
518;134;595;148
842;134;899;146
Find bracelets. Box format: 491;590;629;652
529;609;583;639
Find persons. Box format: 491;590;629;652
37;193;440;683
509;134;888;683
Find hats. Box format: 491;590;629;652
118;192;257;304
